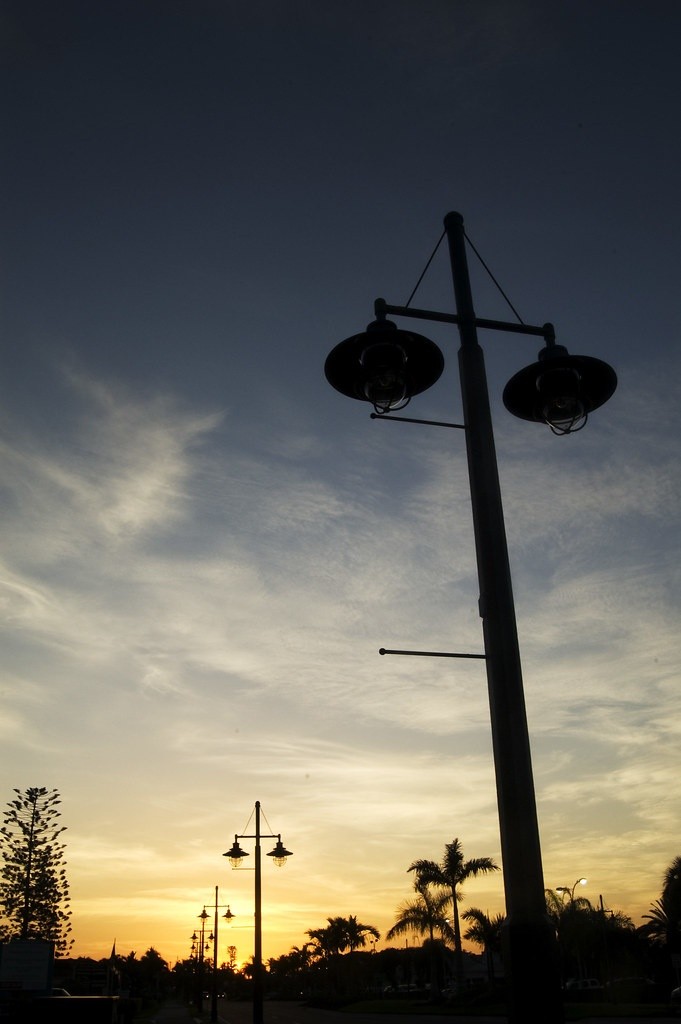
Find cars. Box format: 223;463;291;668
202;991;210;999
564;978;603;993
51;987;71;996
217;992;228;999
670;985;681;1004
601;977;657;1005
379;981;467;996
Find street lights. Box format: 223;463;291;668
319;208;621;1024
187;921;214;1020
222;799;295;1024
197;884;236;1024
